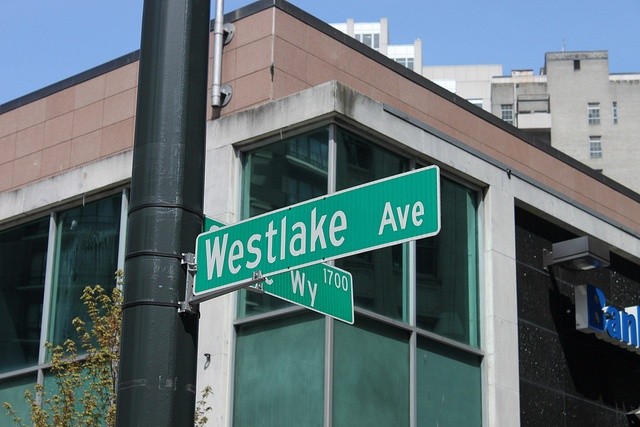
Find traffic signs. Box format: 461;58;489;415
192;165;442;295
246;262;356;325
